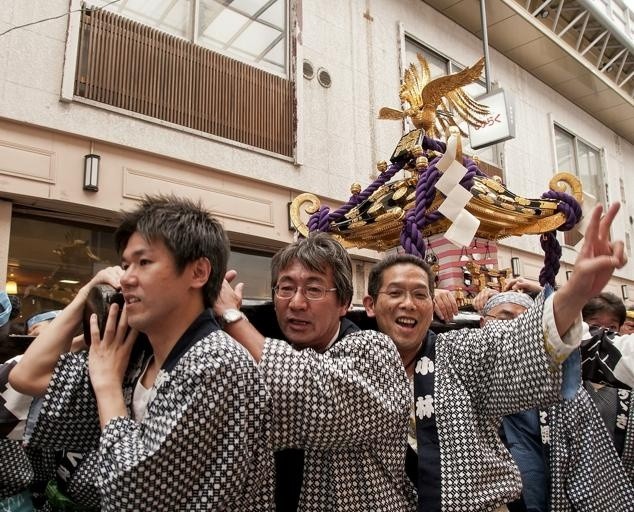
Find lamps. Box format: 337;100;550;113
4;262;19;295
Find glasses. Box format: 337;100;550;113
273;282;339;301
378;287;431;301
485;314;515;321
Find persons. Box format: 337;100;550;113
0;191;277;511
362;200;634;511
211;232;419;512
